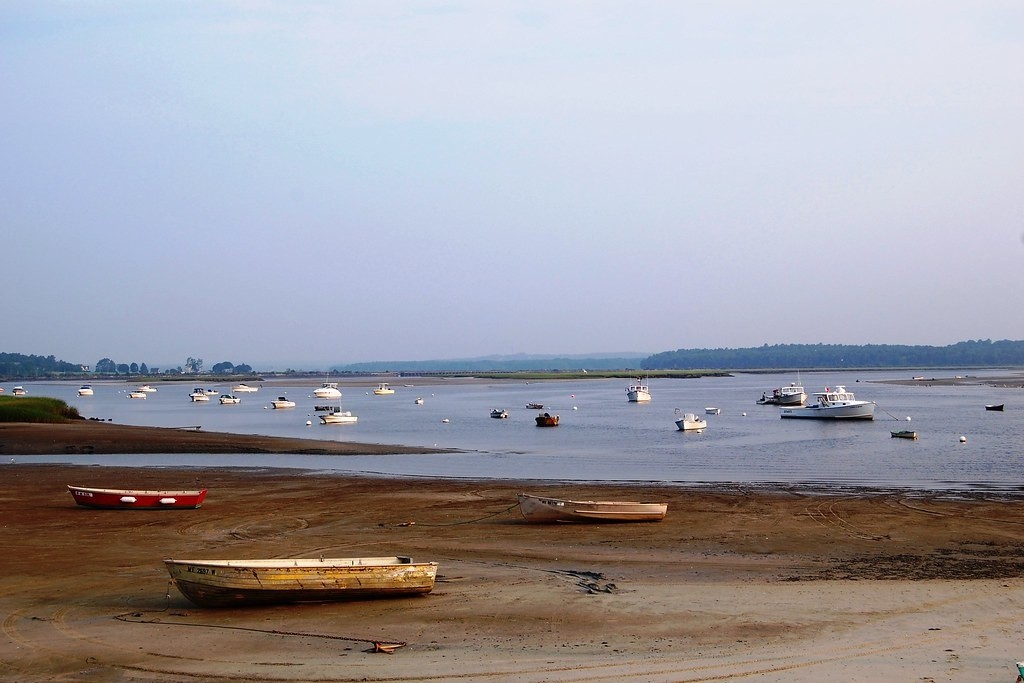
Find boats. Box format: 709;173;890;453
0;381;359;425
913;376;923;379
374;382;395;395
535;413;560;427
756;380;807;406
889;428;917;438
705;407;721;415
404;384;414;387
489;408;508;419
67;483;208;510
526;402;544;409
625;373;652;402
986;402;1006;411
674;413;707;430
162;555;440;608
443;419;449;424
955;376;961;378
515;492;668;523
414;398;424;404
780;384;875;422
